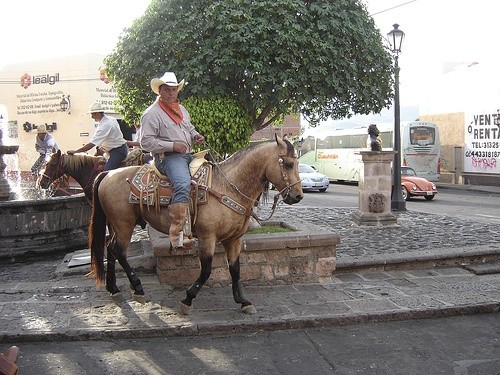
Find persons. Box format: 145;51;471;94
30;124;61;176
140;72;205;250
68;102;129;172
126;120;141;149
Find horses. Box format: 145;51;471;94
87;134;303;316
40;150;155;242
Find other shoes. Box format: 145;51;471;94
3;346;19;364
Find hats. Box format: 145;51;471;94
150;72;184;95
87;103;105;114
36;125;47;134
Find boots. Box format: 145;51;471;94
168;204;196;250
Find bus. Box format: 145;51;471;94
294;119;442;186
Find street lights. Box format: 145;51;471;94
386;22;407;211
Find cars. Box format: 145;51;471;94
267;163;330;193
400;166;439;201
